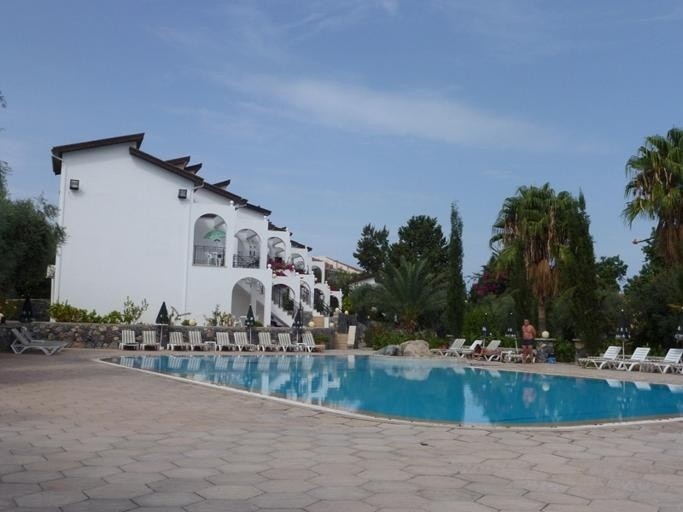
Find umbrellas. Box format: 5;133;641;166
615;309;632;360
504;311;519;354
155;302;170;346
204;229;225;250
482;313;487;347
21;297;32;323
244;305;256;348
292;309;304;342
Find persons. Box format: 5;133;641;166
521;317;537;363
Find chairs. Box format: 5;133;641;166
258;332;277;352
189;331;211;351
463;339;501;361
651;349;682;375
301;333;325;353
449;340;482;358
297;342;304;351
612;347;651;374
211;332;236;351
234;332;255;351
141;330;161;351
167;332;189;352
10;328;61;356
429;338;465;357
278;333;298;352
21;327;68;353
578;345;622;369
118;330;140;350
